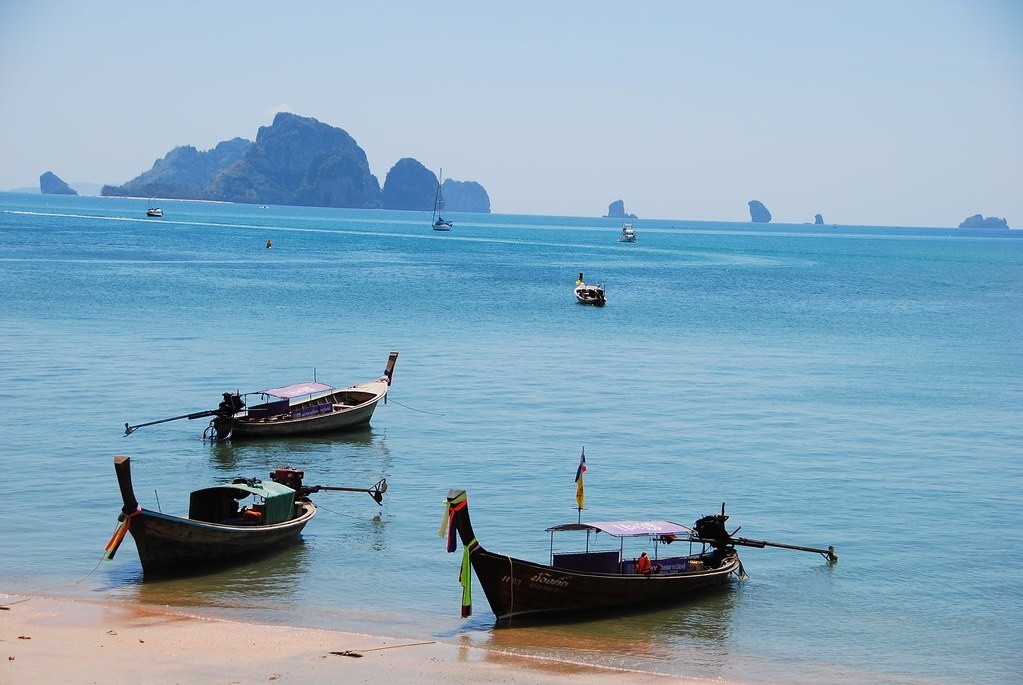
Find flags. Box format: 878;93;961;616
575;450;588;509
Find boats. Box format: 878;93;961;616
112;455;318;578
573;272;606;308
146;207;163;217
202;350;399;442
616;222;636;243
446;487;743;624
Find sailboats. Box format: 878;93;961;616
431;167;453;231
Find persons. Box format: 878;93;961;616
635;551;652;575
